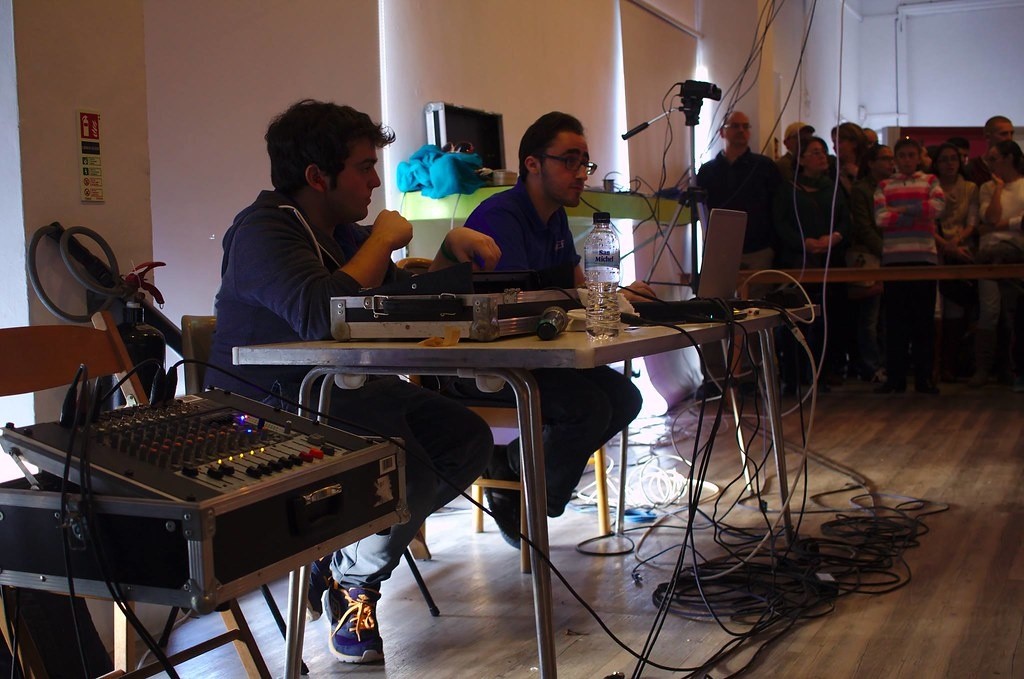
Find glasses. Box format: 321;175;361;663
722;122;752;130
538;152;597;176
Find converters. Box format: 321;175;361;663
814;571;841;595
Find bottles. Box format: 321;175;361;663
584;211;620;342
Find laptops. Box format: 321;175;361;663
697;208;748;301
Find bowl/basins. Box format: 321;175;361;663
484;172;518;186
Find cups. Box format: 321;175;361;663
603;179;614;194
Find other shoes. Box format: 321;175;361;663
967;377;987;387
914;379;940;392
808;383;832;395
680;381;723;409
874;381;906;396
1006;381;1024;394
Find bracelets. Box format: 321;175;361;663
959;234;963;243
441;242;458;263
834;232;842;240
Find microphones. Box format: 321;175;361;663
534;305;568;339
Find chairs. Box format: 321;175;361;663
155;314;440;677
393;258;612;570
0;312;271;679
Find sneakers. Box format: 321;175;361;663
324;577;384;663
305;553;335;623
509;439;564;520
479;444;521;550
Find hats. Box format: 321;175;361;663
784;122;815;141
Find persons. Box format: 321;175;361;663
778;116;1024;393
202;98;502;664
697;110;786;404
463;112;656;548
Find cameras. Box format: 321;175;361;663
679;79;722;101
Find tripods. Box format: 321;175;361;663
622;96;774;496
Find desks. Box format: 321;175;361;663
677;258;1024;410
231;301;819;679
